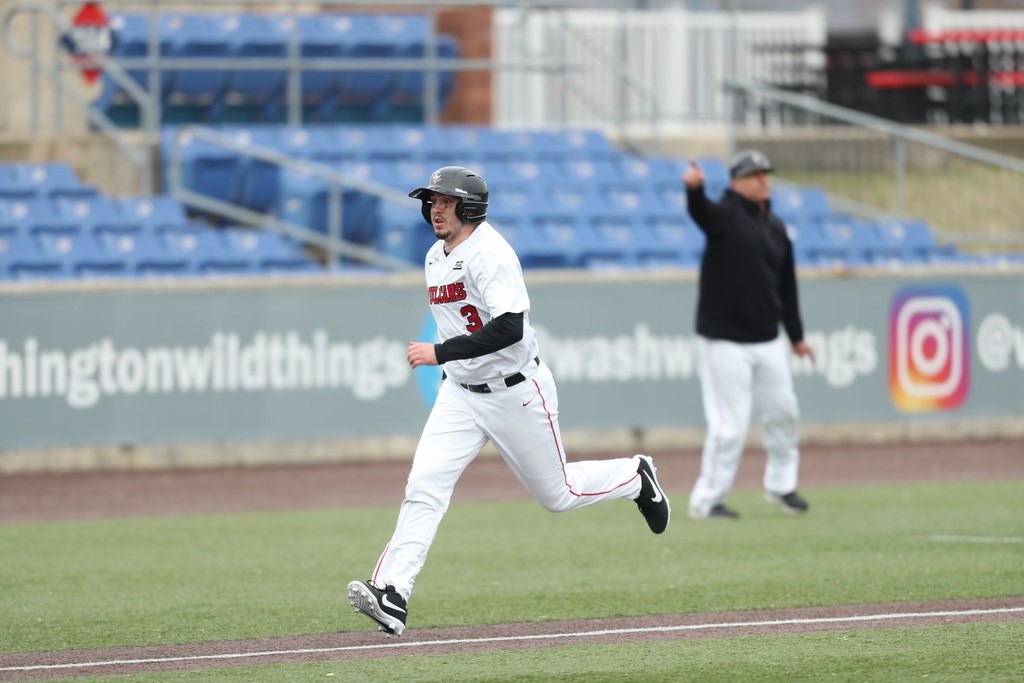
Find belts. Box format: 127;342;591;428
461;356;541;393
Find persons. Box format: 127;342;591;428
681;148;816;519
348;166;672;637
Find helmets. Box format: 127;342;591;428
408;166;488;225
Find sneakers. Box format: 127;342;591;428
709;504;737;518
348;579;408;639
765;491;807;515
633;454;672;534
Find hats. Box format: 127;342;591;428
729;150;775;178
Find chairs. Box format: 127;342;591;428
0;11;1023;278
